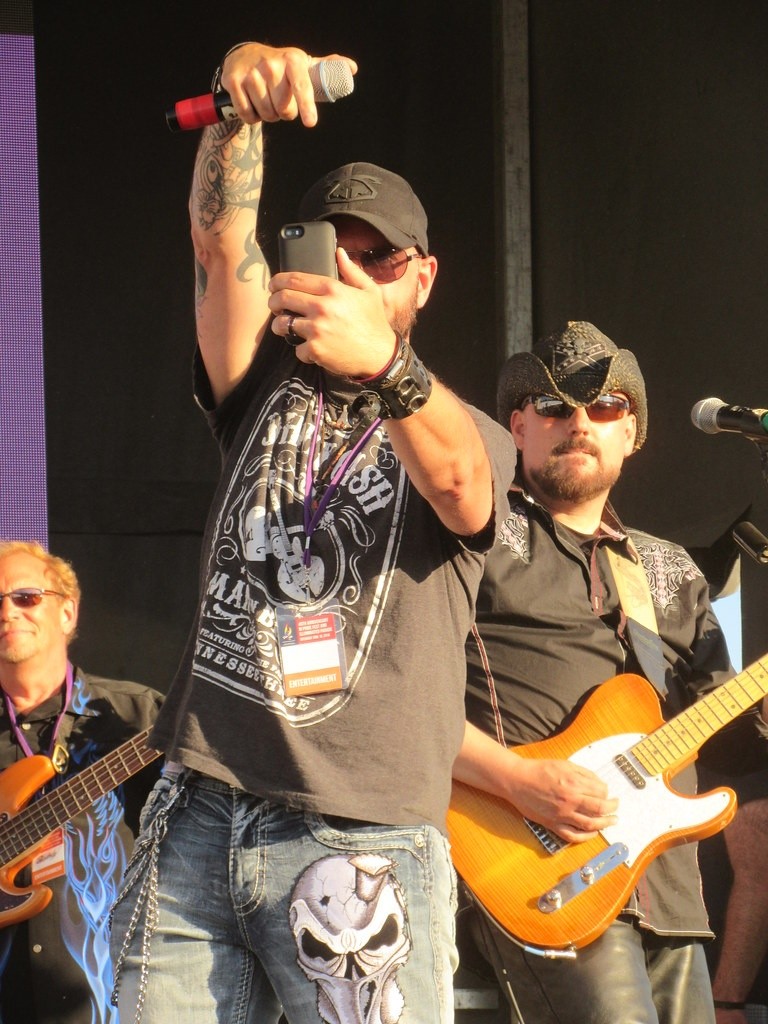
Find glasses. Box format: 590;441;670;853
331;243;424;282
0;587;68;608
519;392;634;422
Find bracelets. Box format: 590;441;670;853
713;1000;744;1010
348;331;432;419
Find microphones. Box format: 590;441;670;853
691;398;768;440
165;60;355;132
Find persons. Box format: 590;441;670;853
451;319;768;1024
0;541;173;1024
104;43;519;1024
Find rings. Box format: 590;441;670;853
288;316;295;334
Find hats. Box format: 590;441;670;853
297;161;431;256
496;322;646;453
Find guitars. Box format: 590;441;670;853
0;720;166;927
449;654;768;963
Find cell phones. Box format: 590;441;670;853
278;222;339;282
